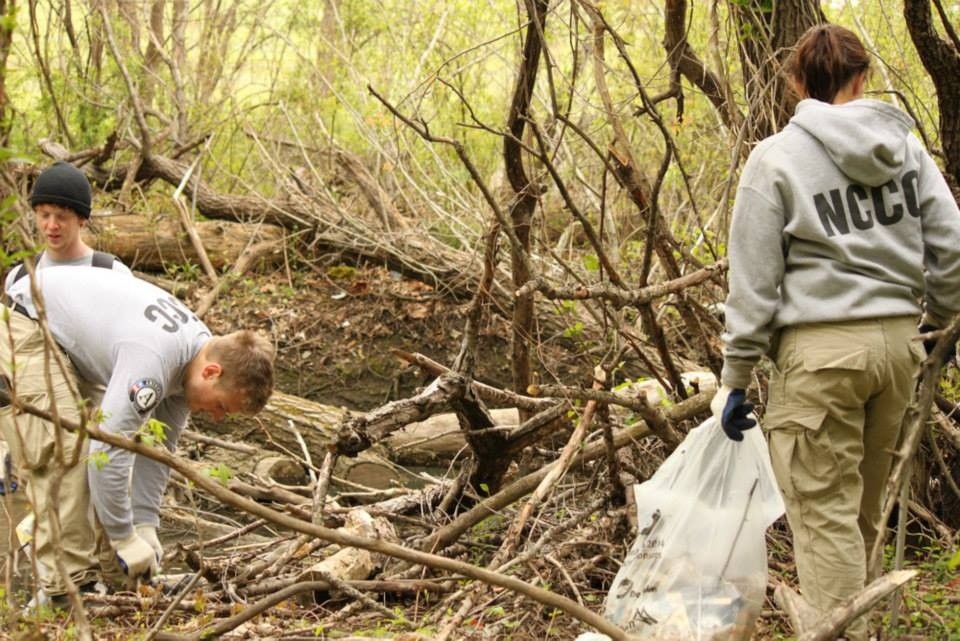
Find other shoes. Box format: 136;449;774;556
51;580;118;604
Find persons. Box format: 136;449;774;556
4;164;134;291
720;23;960;641
0;266;274;615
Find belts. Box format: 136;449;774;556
1;292;26;315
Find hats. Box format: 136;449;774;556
30;160;92;219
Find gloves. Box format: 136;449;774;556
134;524;165;584
108;525;158;581
709;384;758;443
918;322;957;365
